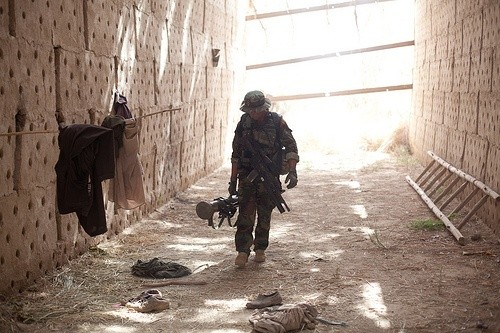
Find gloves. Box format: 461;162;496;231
285;169;298;189
228;181;237;194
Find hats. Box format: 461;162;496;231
240;90;271;112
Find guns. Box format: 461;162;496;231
239;133;290;214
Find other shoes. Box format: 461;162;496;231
235;251;249;268
126;288;164;309
246;291;283;307
252;247;267;261
137;297;169;313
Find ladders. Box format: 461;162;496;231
404;149;500;242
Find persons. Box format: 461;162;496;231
226;90;300;268
